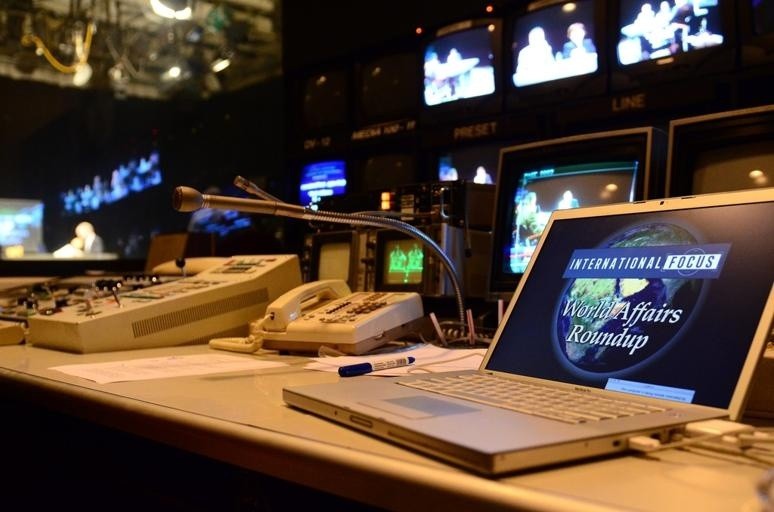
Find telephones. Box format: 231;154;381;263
249;279;425;356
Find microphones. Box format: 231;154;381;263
234;175;284;203
172;184;306;220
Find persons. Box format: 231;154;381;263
446;47;464;83
558;191;580;210
518;190;541;215
516;26;556;72
425;52;442;80
561;19;594;58
70;220;106;255
622;2;722;53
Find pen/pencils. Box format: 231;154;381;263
338;357;415;378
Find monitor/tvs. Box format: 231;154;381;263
60;146;169;220
294;152;358;208
485;124;666;303
373;221;492;301
662;103;774;198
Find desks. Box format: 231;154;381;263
1;342;774;512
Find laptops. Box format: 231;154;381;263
281;187;774;478
